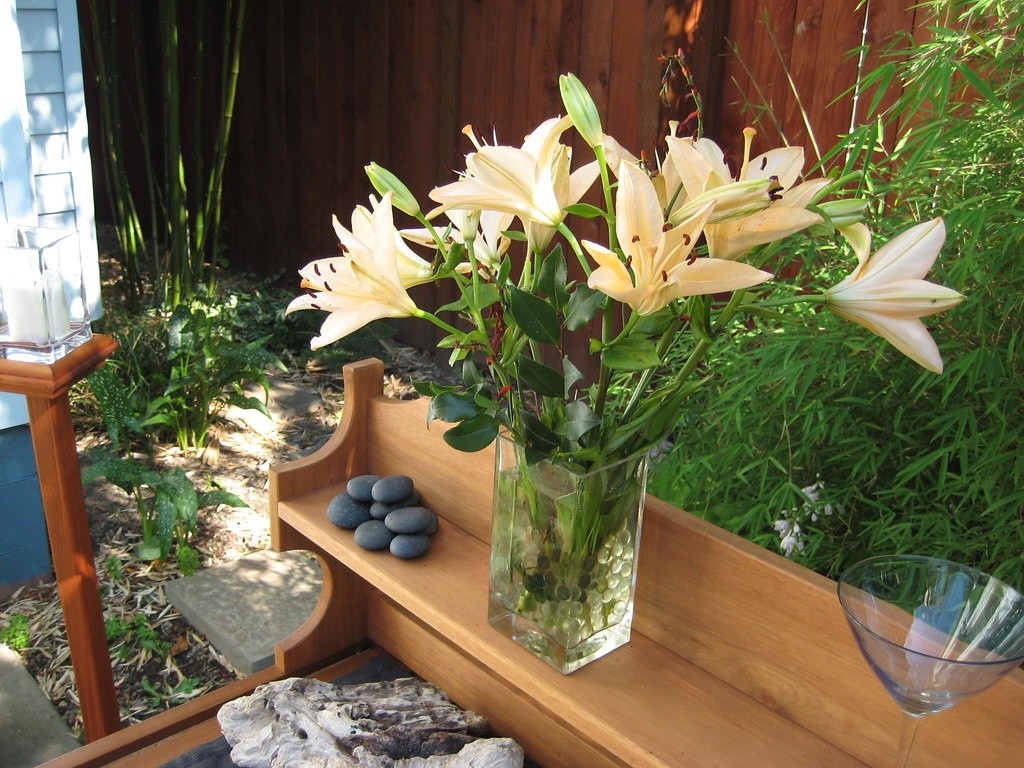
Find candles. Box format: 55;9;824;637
0;224;93;365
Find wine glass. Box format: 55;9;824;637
837;554;1024;768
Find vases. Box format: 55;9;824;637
489;423;651;676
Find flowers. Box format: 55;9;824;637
465;500;477;503
285;74;967;623
